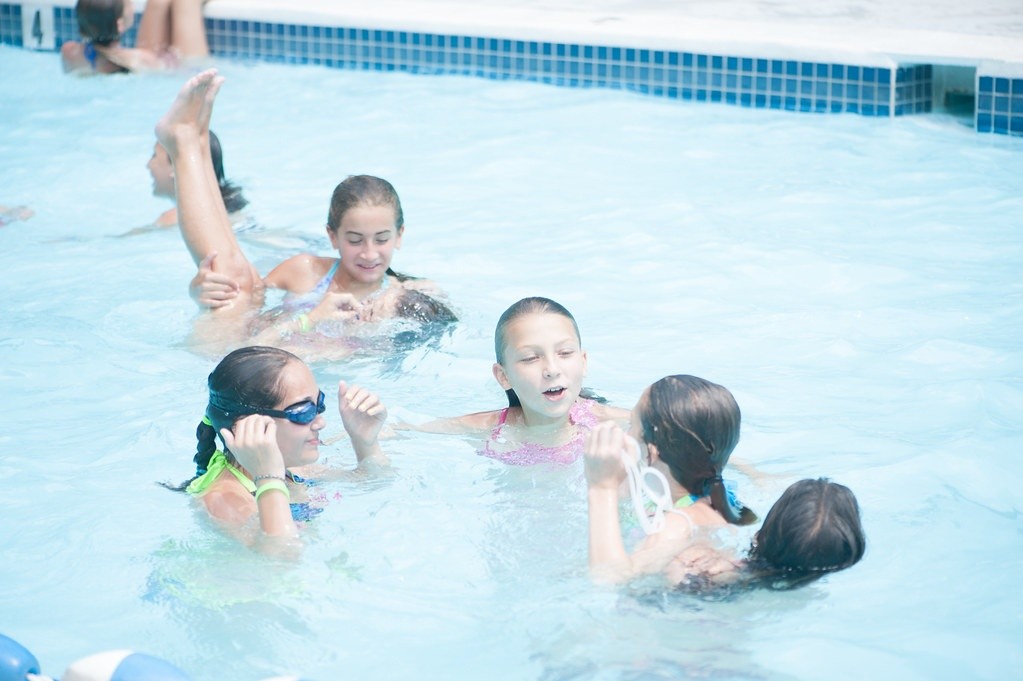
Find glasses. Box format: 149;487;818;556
620;436;672;534
210;388;327;424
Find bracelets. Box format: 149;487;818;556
254;475;290;487
298;312;310;333
255;483;291;506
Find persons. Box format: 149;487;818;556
113;128;247;239
152;347;388;569
589;374;757;593
152;70;457;362
187;175;441;337
318;297;767;482
63;1;215;79
664;478;866;602
